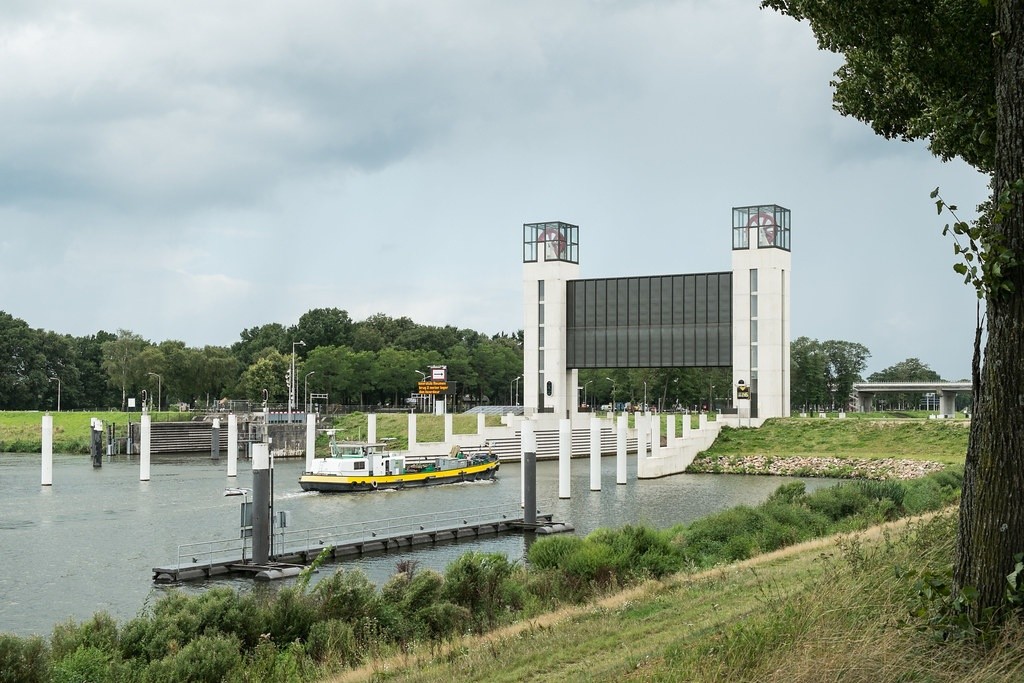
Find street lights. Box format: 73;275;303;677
50;377;60;413
415;370;426;414
148;372;161;412
284;368;293;424
420;376;431;410
585;380;593;408
510;376;521;406
291;340;307;411
305;371;316;413
606;377;616;411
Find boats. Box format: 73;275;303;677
297;428;501;494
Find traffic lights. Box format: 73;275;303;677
262;389;269;401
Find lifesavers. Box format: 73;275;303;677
496;465;499;471
425;477;429;483
486;468;490;474
351;481;357;488
361;481;365;487
372;480;377;488
